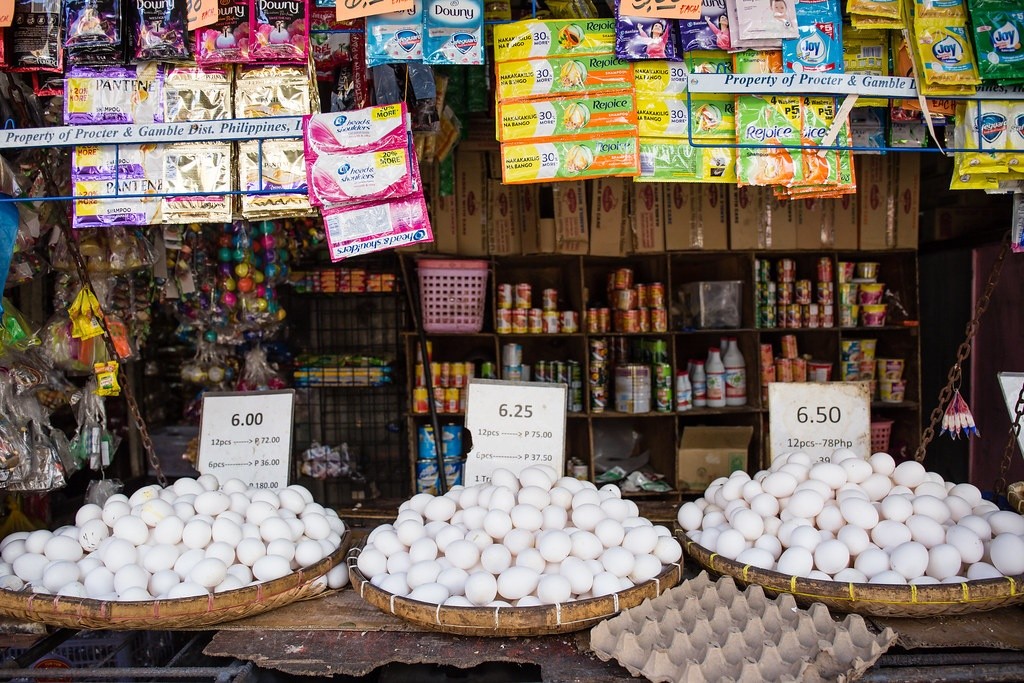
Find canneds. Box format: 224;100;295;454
502;344;584;413
754;256;835;329
589;337;673;414
586;268;667;333
761;335;807;407
413;341;496;414
566;455;588;481
497;283;579;334
416;424;463;496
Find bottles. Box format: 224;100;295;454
675;336;748;413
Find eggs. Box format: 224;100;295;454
357;465;682;608
678;447;1024;584
0;474;349;595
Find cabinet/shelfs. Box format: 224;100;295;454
124;250;408;522
400;248;924;500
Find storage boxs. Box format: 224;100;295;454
433;119;1001;253
0;631;189;683
145;426;198;478
678;424;755;492
673;279;744;329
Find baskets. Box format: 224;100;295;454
673;505;1024;618
348;534;685;637
415;258;489;333
0;517;350;630
